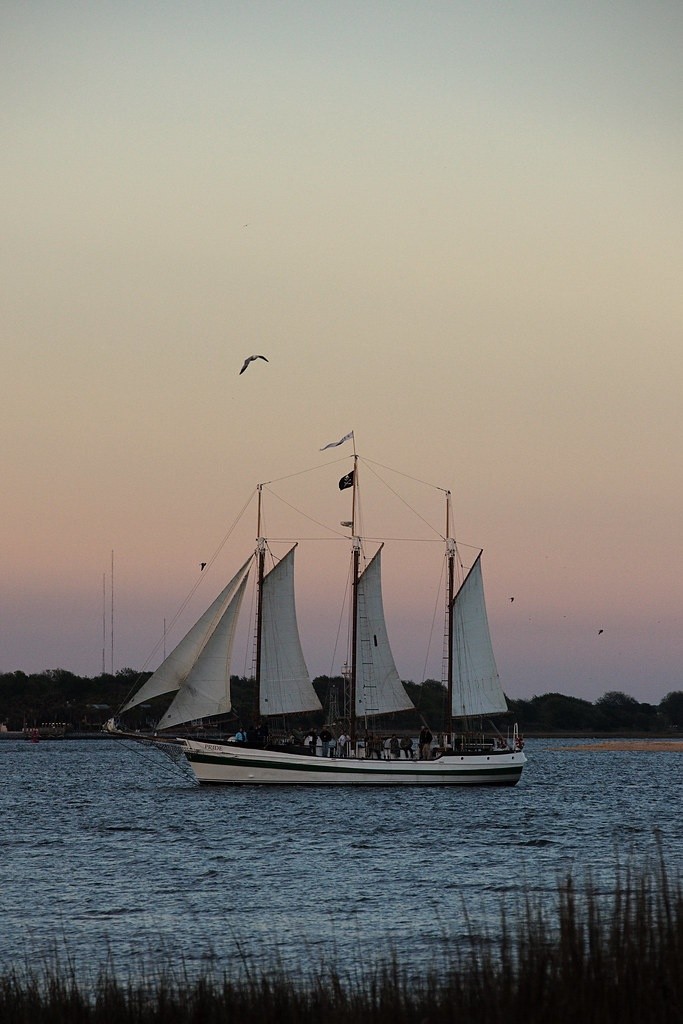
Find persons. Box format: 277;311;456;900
236;722;268;742
339;731;351;758
320;727;331;756
390;734;401;758
306;728;318;755
419;726;433;760
400;733;413;758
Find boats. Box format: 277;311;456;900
0;718;74;739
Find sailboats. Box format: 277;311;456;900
100;430;527;789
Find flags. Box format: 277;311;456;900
339;471;354;490
320;432;353;451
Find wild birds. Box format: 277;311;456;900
237;354;270;376
